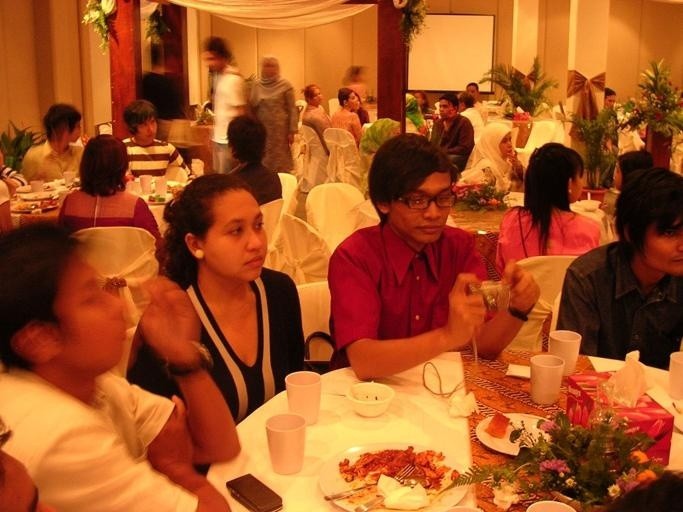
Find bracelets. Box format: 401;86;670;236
30;486;39;512
505;304;528;321
163;365;199;377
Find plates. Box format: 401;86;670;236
476;413;561;456
11;178;79;213
150;181;189;192
319;443;469;512
140;193;174;205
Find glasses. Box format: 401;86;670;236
397;191;458;209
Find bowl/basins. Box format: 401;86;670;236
581;200;602;212
345;383;395;417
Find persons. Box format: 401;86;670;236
2;37;682;278
557;168;683;369
602;471;683;511
1;220;232;512
1;418;58;512
127;172;303;472
329;132;541;382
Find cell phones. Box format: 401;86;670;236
226;472;284;512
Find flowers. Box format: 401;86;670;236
620;58;683;161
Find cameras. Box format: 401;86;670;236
467;278;515;311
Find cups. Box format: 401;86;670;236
530;355;565;405
139;175;152;194
549;330;582;376
155;176;167;196
667;352;683;400
285;371;321;426
265;413;306;475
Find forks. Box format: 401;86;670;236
325;464;416;502
